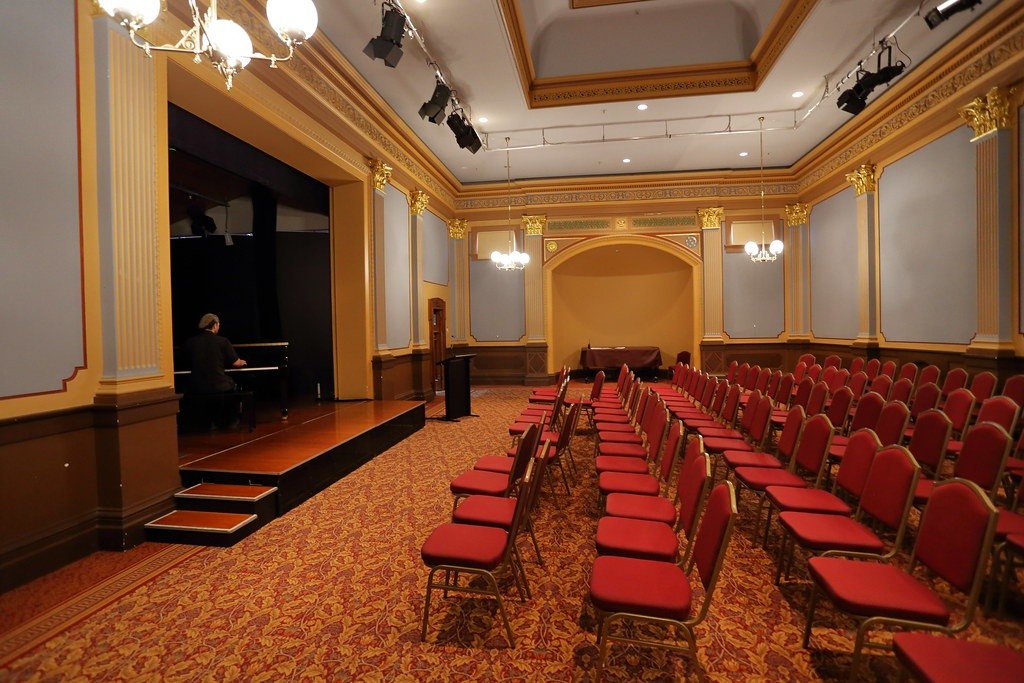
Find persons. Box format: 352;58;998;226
185;313;247;430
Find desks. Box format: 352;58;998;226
182;391;256;433
580;346;662;382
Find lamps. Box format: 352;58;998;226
837;34;913;115
923;0;983;31
94;0;318;93
363;10;406;69
743;117;784;263
490;137;530;271
418;73;482;155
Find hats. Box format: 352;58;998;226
199;313;219;329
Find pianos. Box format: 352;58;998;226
172;335;294;420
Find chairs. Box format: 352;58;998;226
421;352;1024;683
